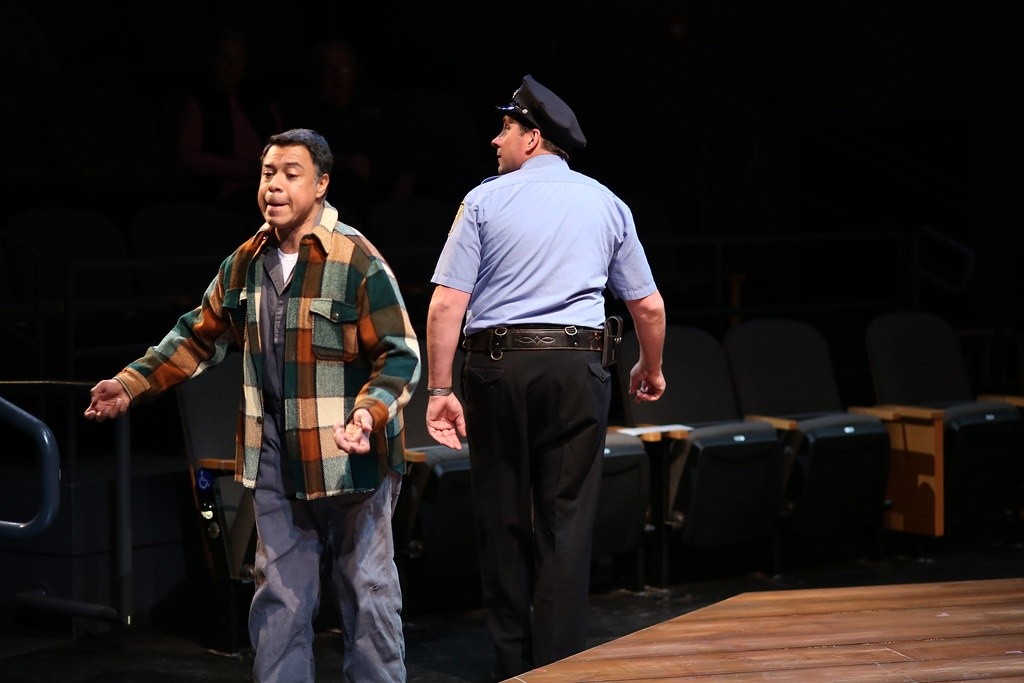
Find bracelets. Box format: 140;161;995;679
427;386;454;396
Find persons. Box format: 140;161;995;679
425;76;666;683
83;130;421;683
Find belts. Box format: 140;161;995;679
466;327;614;353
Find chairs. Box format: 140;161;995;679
173;308;1024;656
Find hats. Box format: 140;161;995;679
499;74;587;150
